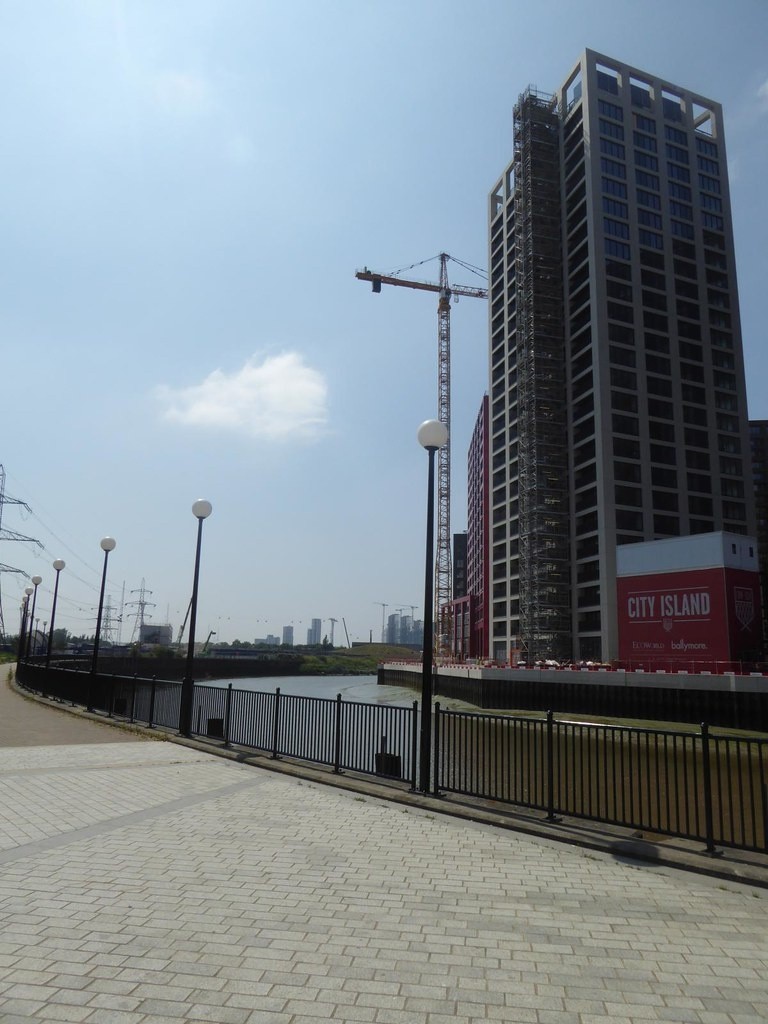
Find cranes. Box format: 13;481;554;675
394;603;418;621
373;600;388;644
355;250;491;669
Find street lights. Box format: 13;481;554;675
84;535;117;713
180;498;213;738
414;418;448;795
42;620;47;653
21;575;43;686
17;586;34;661
32;617;40;655
41;558;66;698
